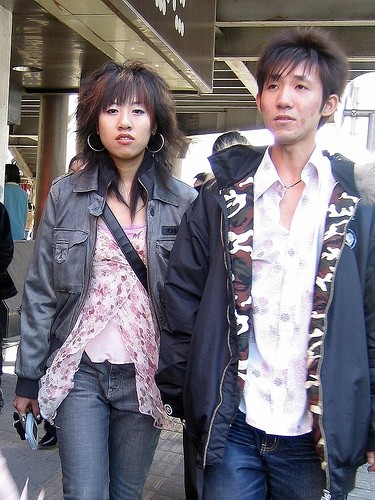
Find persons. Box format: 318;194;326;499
156;43;374;496
68;150;89;173
205;129;250;163
190;170;215;189
12;58;200;495
6;160;29;242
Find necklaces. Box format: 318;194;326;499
274;182;291;197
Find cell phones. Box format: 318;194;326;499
25;410;38;451
